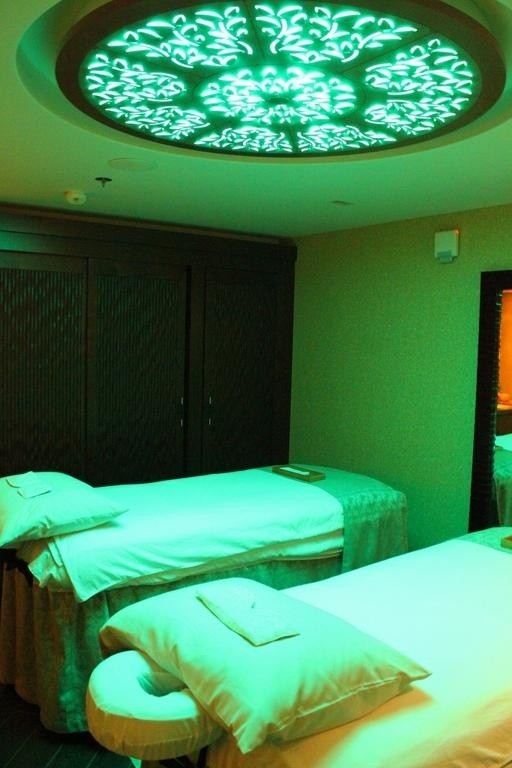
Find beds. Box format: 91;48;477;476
4;464;407;734
86;525;512;767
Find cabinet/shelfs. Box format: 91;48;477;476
0;201;297;487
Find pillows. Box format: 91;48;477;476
0;472;129;549
99;576;433;756
